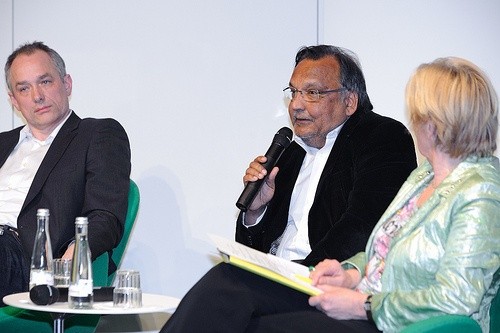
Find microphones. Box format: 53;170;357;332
30;284;127;306
236;126;293;212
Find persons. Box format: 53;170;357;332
235;44;417;333
0;40;131;306
158;57;500;333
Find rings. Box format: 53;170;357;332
309;266;315;271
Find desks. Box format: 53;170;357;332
2;291;181;333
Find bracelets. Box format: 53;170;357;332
365;296;373;321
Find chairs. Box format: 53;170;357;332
0;177;141;333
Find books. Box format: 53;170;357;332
219;252;319;296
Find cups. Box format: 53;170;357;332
52;258;72;287
112;270;142;310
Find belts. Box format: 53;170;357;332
0;226;19;235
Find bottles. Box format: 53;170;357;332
27;208;56;292
66;216;95;310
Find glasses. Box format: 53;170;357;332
282;87;348;102
